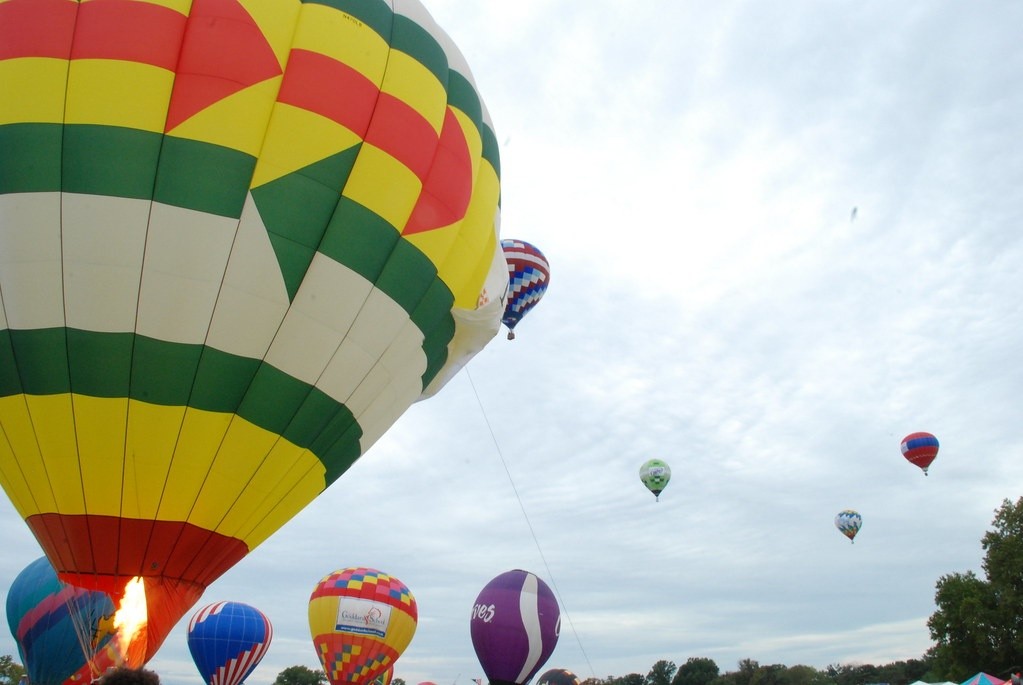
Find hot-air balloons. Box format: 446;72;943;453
4;555;129;685
834;509;862;543
187;599;273;685
469;569;561;685
307;566;418;685
500;237;550;342
900;432;939;476
536;668;581;685
638;459;670;501
0;0;501;685
